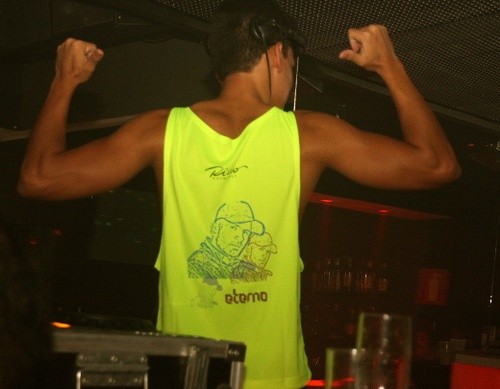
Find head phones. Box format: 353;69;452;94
205;16;307;57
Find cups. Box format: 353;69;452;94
356;312;412;388
325;347;389;389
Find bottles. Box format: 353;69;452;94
342;309;357;346
318;256;392;299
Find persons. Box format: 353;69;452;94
13;0;462;389
